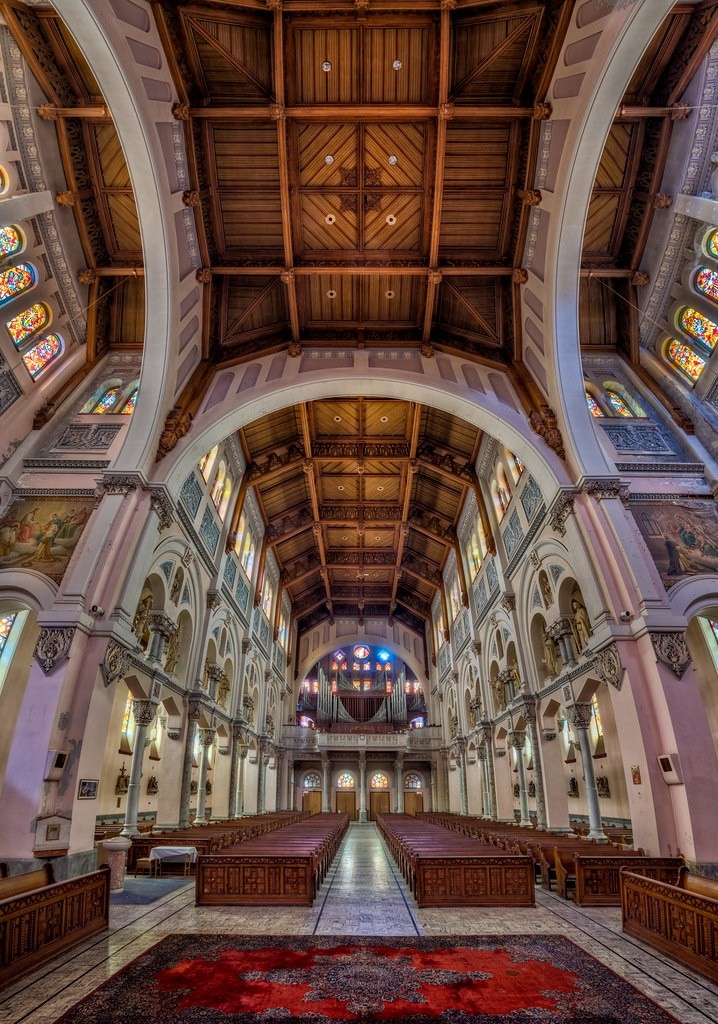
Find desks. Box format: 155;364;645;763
149;846;198;878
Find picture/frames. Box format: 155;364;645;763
77;778;100;800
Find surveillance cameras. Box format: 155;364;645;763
619;611;630;617
91;605;104;615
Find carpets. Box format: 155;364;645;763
109;878;196;905
53;934;685;1024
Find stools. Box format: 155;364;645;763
134;858;155;878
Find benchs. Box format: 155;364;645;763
0;863;56;900
673;866;718;900
95;810;647;900
